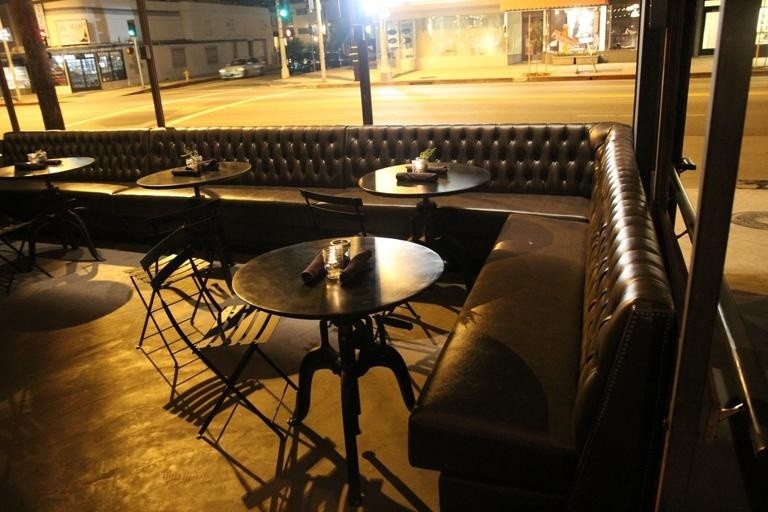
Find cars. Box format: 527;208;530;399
219;56;264;80
287;52;345;73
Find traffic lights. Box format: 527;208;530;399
127;20;137;37
279;0;292;24
125;47;134;54
283;27;296;38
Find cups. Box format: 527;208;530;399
412;159;425;174
321;238;351;282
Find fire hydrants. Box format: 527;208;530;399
183;70;190;80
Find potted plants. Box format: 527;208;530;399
552;52;600;65
